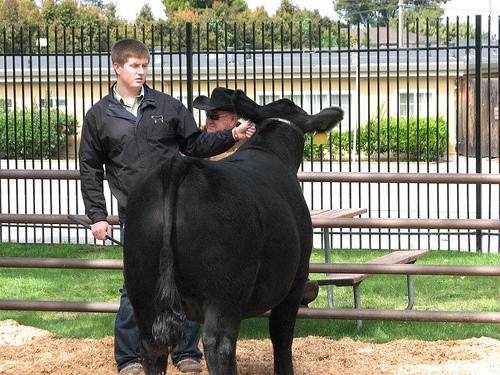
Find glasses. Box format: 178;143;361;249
206;111;229;120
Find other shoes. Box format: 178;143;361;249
120;363;143;375
300;280;319;308
176;358;202;373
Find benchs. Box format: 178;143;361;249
317;250;428;286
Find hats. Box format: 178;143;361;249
193;87;235;111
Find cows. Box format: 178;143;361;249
122;90;344;375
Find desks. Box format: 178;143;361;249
306;208;368;308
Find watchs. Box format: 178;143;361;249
234;126;239;141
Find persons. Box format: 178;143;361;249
79;39;256;375
193;86;319;309
